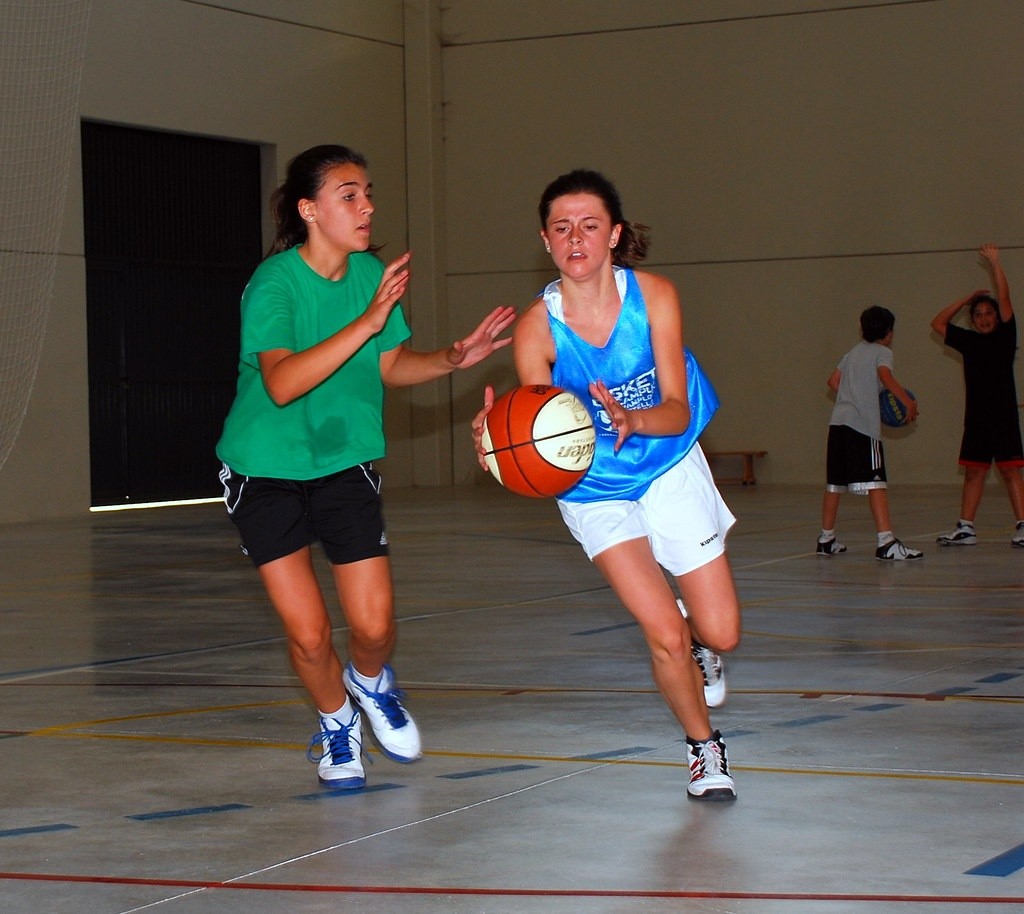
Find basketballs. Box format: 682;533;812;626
481;384;596;499
879;388;916;427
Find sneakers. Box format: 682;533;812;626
343;663;422;764
1011;522;1024;547
876;539;925;560
684;732;737;800
936;522;978;544
318;708;368;789
691;642;727;708
817;535;848;555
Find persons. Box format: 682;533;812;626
812;304;927;561
931;241;1022;555
214;140;517;791
468;169;745;802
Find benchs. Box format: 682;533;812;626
700;451;769;486
